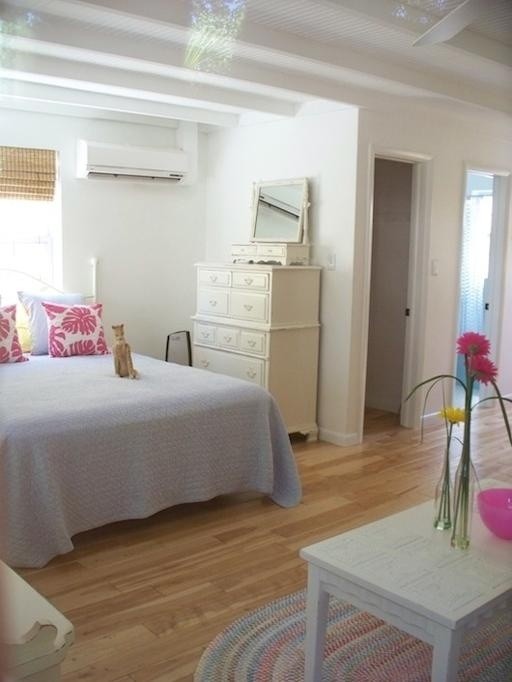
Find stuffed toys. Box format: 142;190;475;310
110;323;138;379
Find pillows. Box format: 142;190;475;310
0;289;113;362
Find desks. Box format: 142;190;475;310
299;473;511;682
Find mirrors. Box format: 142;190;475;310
249;178;308;244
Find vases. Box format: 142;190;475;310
452;444;479;550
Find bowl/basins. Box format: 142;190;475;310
477;489;511;541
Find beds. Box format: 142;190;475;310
1;257;302;570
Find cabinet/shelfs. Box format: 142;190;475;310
231;243;310;265
192;265;324;442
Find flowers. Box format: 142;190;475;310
403;331;512;542
182;0;246;72
0;10;41;70
393;0;457;27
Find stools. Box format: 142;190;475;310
1;561;74;681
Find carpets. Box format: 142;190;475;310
195;588;511;681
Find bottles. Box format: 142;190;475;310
110;322;135;378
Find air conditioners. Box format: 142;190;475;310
259;193;300;221
75;139;189;188
